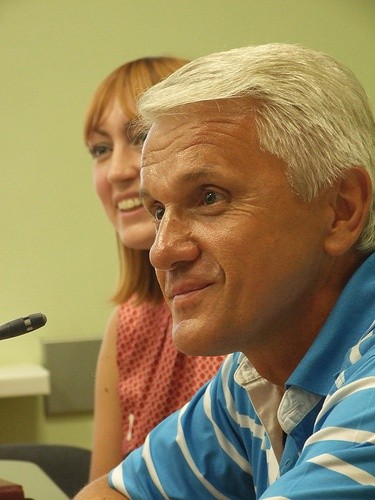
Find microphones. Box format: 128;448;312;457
0;313;47;340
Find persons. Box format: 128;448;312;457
84;57;229;483
74;43;374;500
